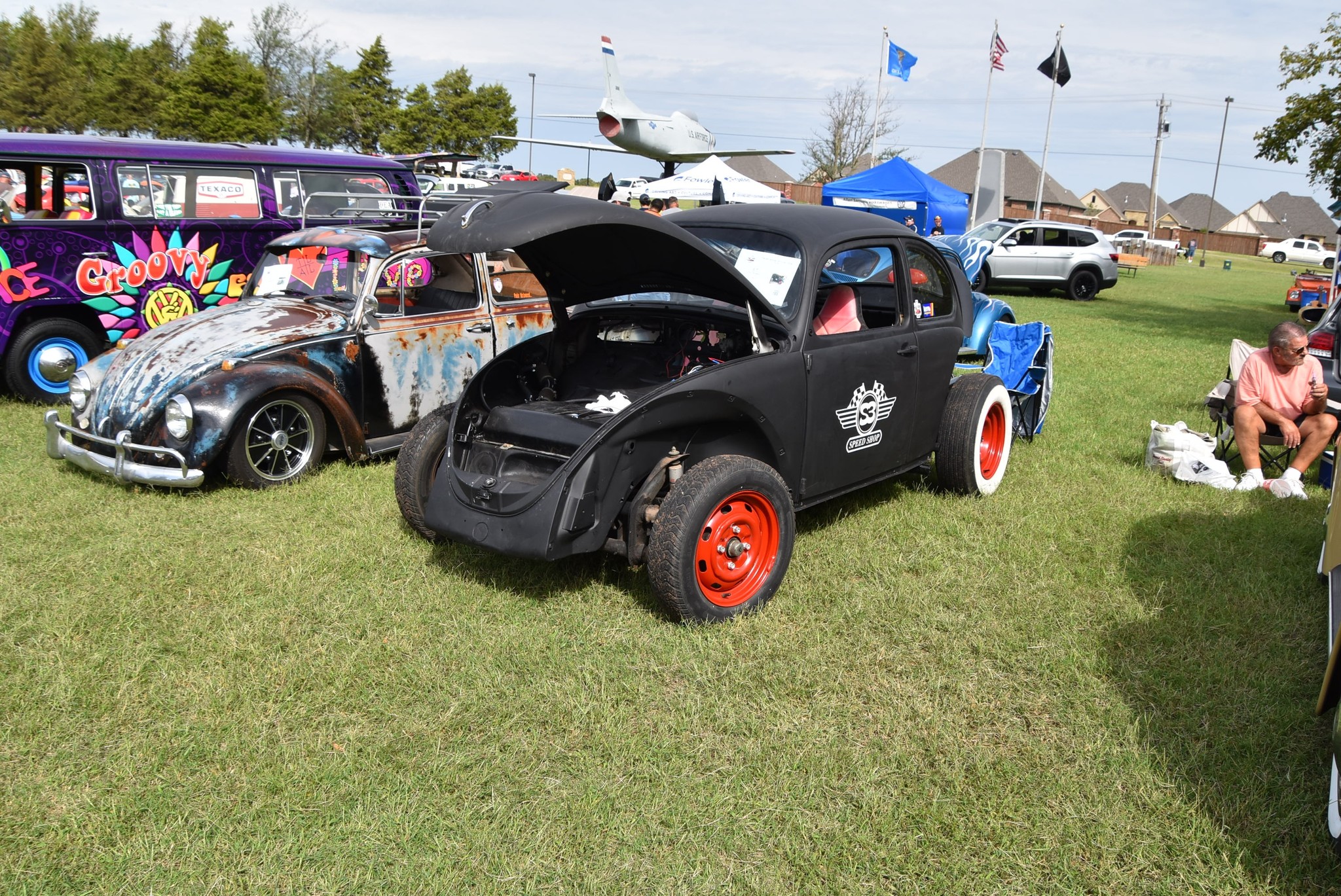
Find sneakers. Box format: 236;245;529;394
1233;472;1264;491
1279;474;1309;501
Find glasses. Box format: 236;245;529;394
1272;341;1310;355
906;220;911;222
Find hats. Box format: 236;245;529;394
934;216;941;220
0;172;11;178
903;216;914;220
640;194;650;201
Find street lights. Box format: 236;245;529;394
527;72;537;181
1199;96;1234;268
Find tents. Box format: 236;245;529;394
821;156;969;237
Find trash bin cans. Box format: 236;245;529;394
1335;271;1341;285
1224;260;1232;269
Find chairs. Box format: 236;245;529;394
1199;338;1341;477
23;208;59;220
811;282;871;335
59;209;92;220
950;321;1056;448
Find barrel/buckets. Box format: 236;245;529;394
1301;289;1327;309
1223;261;1231;269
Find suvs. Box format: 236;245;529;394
960;217;1119;300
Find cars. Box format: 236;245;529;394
1284;267;1341;313
45;160;1018;624
1259;238;1337;269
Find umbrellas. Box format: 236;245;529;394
628;154;781;203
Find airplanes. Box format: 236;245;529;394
489;84;796;180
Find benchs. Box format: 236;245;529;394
1117;253;1149;279
414;285;530;311
377;301;455;316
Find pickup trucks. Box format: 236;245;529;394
1103;230;1187;255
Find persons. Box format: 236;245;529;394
903;215;917;233
607;191;631;207
1188;238;1197;263
637;194;682;217
122;173;141;205
930;216;944;237
1234;323;1337;500
0;171;18;213
1175;238;1183;256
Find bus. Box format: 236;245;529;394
0;131;427;405
